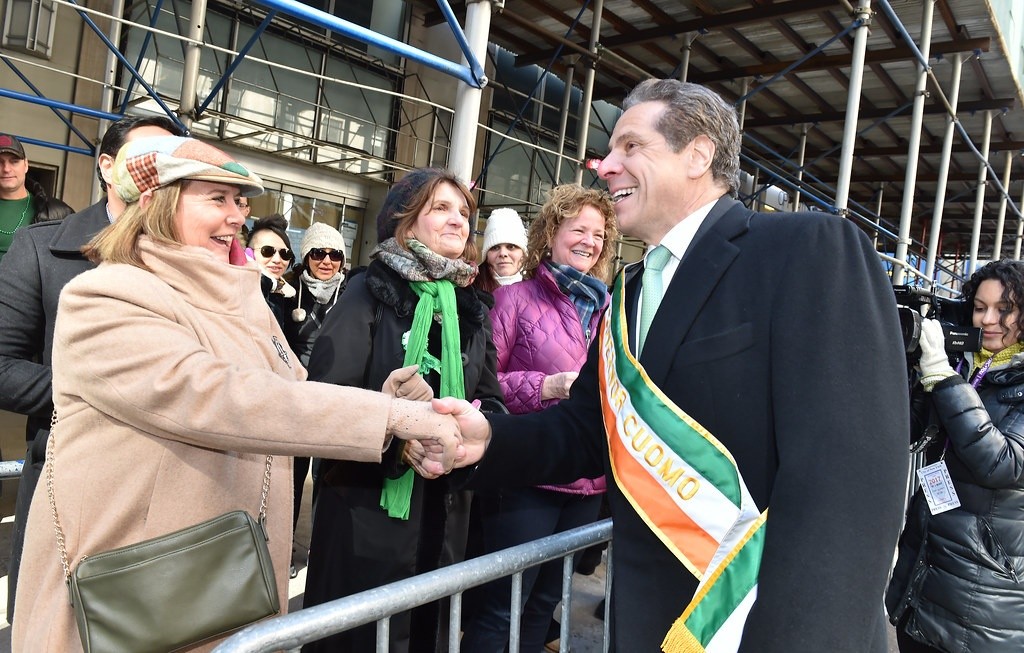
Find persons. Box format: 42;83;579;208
884;258;1024;653
423;79;910;653
0;115;614;653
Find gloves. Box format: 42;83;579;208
917;319;959;392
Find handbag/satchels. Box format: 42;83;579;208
65;510;281;653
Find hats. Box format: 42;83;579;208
112;135;265;203
482;208;529;262
292;222;346;322
0;134;25;159
376;170;439;251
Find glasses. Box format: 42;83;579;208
252;246;293;260
308;248;343;261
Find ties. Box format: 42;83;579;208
638;245;672;361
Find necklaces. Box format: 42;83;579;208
0;188;31;235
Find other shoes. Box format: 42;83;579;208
543;618;571;653
